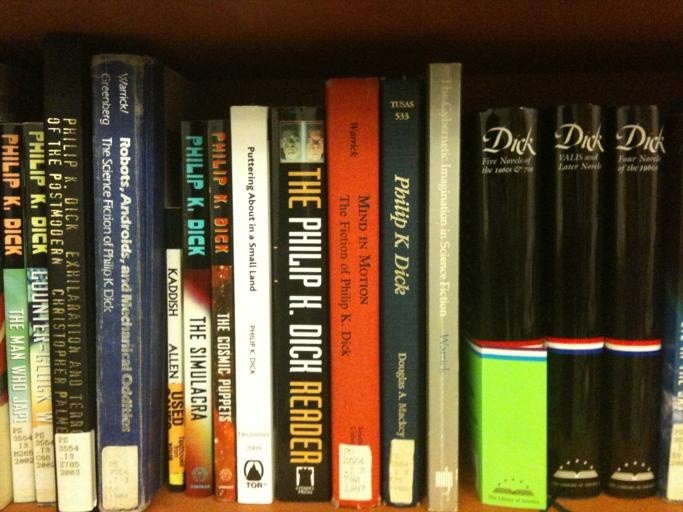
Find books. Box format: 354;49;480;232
0;31;683;510
